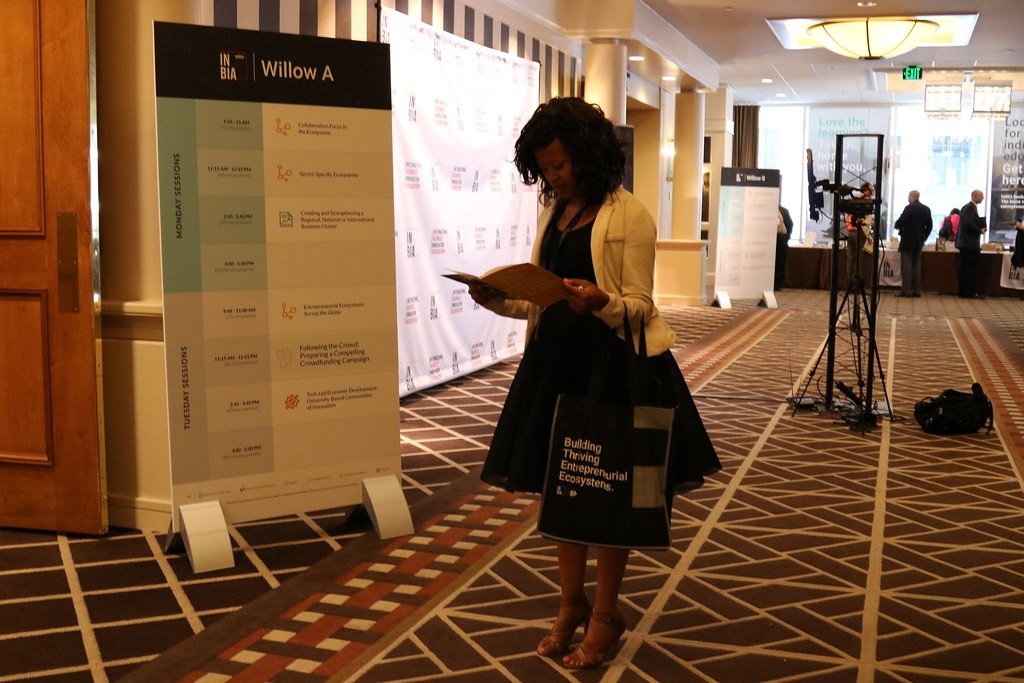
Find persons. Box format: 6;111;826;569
468;97;680;669
845;183;875;294
1011;215;1024;267
939;208;960;242
893;190;933;298
774;205;794;291
957;189;987;299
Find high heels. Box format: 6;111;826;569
537;599;594;657
563;610;626;669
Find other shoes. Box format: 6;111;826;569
912;291;921;297
894;292;910;298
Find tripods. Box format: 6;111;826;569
790;215;895;423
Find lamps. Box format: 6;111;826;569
805;18;940;60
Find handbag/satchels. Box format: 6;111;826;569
939;216;952;237
913;384;993;435
777;209;787;235
977;217;987;230
537;299;674;551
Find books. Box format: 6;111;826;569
440;262;569;307
